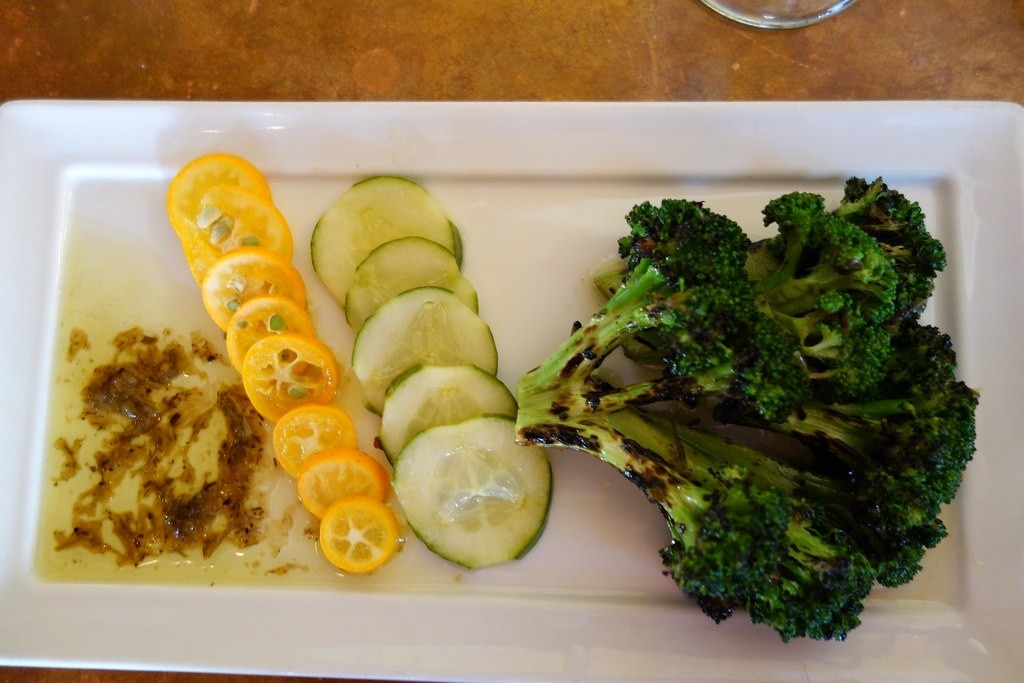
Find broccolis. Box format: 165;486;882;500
517;177;979;644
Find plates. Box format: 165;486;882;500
0;97;1023;683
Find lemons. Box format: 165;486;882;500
167;156;395;572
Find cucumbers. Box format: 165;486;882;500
312;176;551;569
